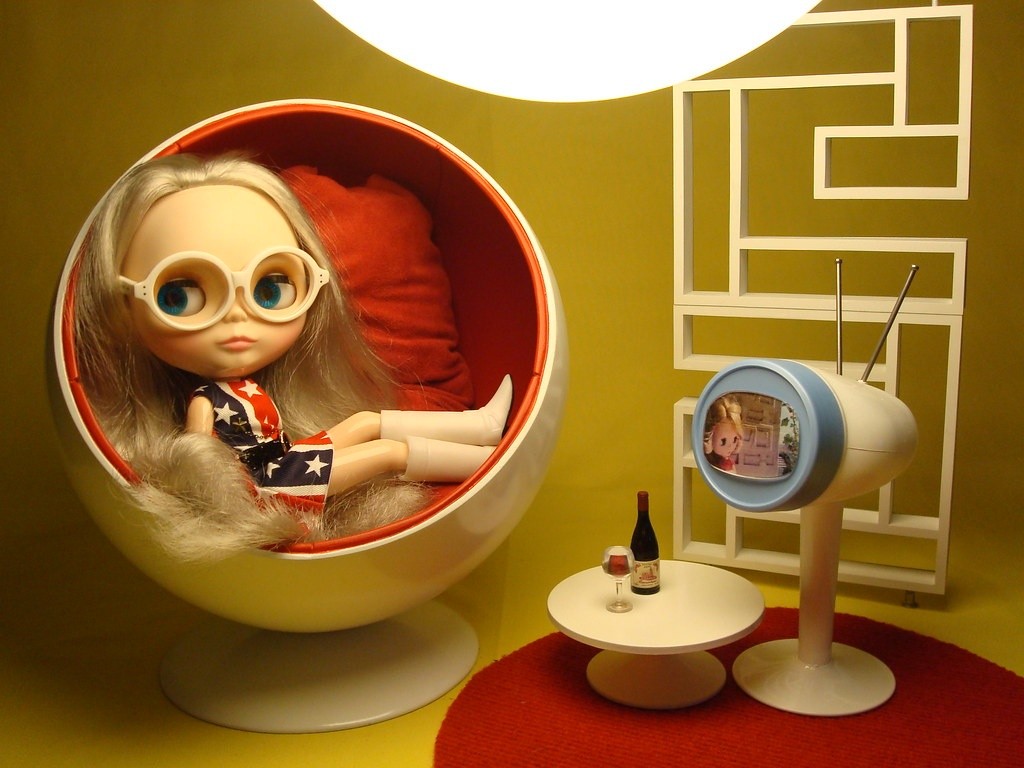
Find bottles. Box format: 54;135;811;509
631;491;660;595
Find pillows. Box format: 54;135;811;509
280;167;471;412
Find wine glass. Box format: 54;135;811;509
602;545;635;613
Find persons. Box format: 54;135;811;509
702;393;744;475
71;149;514;561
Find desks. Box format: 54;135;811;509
544;557;766;708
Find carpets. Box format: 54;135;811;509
429;607;1024;767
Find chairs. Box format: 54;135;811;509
42;98;563;737
